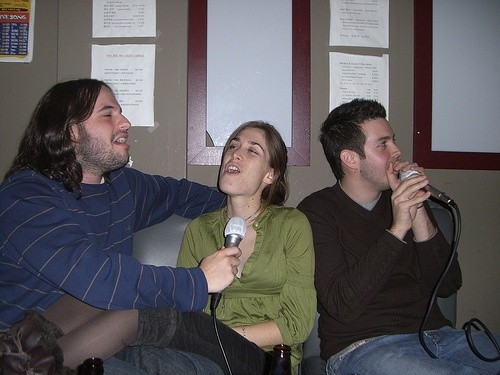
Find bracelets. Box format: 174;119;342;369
241;326;246;338
417;204;424;209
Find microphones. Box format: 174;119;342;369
209;216;248;310
398;169;456;211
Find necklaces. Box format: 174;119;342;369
228;205;262;222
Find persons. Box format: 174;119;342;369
0;78;242;375
296;98;500;375
0;121;314;375
